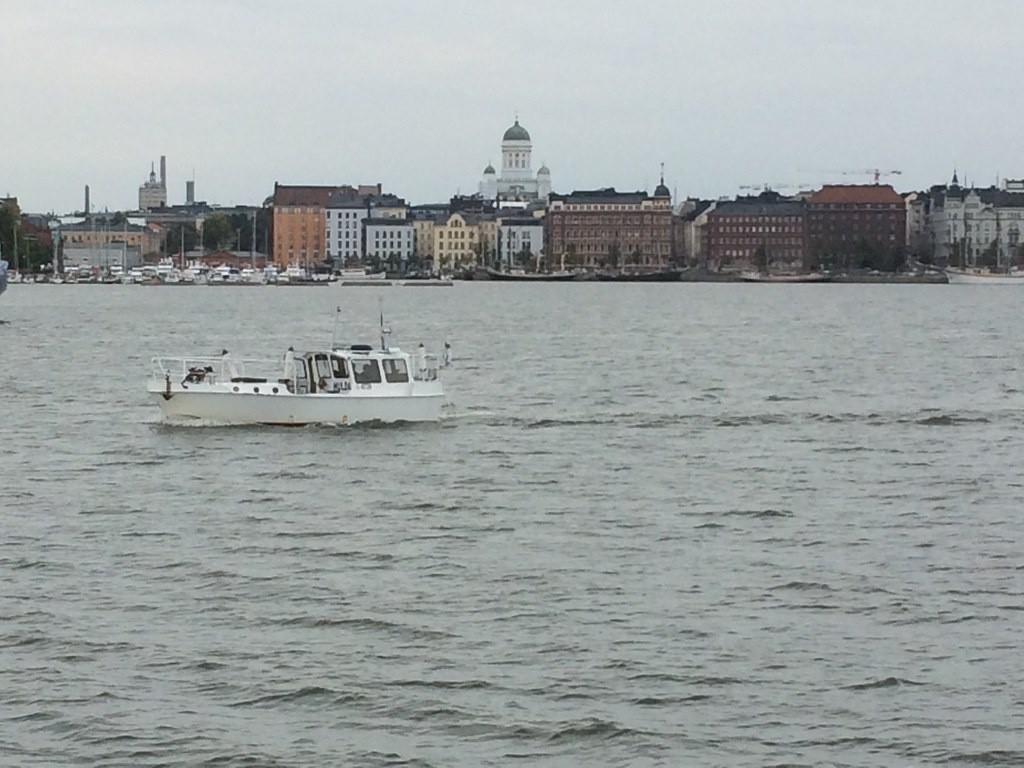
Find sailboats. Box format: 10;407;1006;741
6;211;306;286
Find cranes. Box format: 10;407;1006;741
739;168;901;191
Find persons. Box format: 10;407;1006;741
352;363;380;382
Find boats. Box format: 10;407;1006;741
486;266;577;282
734;270;831;283
146;306;452;426
595;270;684;282
